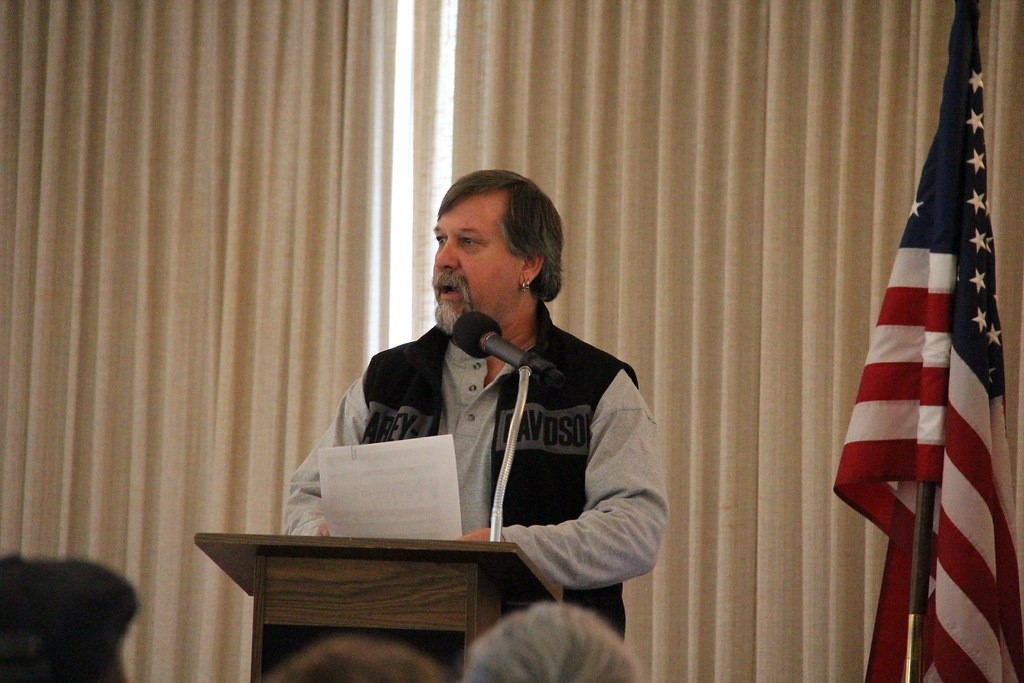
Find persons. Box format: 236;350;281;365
279;167;671;645
257;603;647;683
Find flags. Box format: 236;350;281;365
832;2;1024;682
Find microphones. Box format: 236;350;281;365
453;311;565;388
